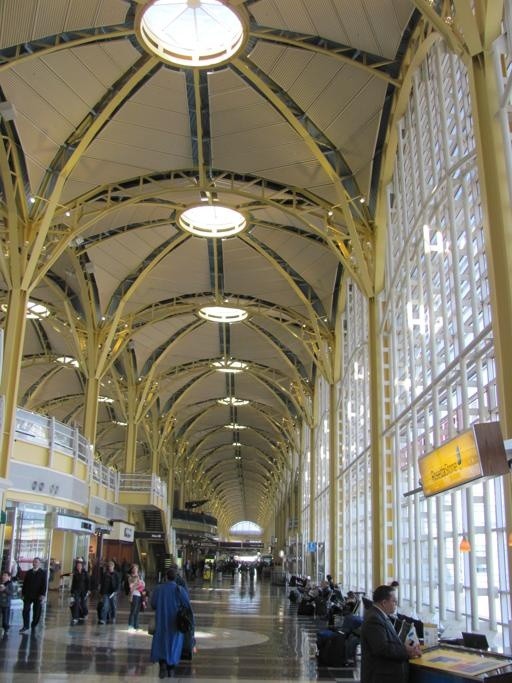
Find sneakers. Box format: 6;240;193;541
159;664;174;678
19;624;35;632
73;615;88;624
100;618;115;624
128;626;143;632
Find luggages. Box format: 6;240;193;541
317;628;358;666
181;633;193;660
70;600;85;618
97;602;109;621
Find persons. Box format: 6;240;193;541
124;562;146;631
319;590;356;630
389;580;400;616
1;623;150;682
150;566;197;679
169;563;190;599
122;557;129;572
360;583;423;682
20;555;48;632
69;562;91;625
185;558;262;581
1;570;15;636
296;579;332;616
97;560;121;623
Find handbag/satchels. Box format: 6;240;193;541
148;617;155;635
177;606;193;633
128;592;133;603
